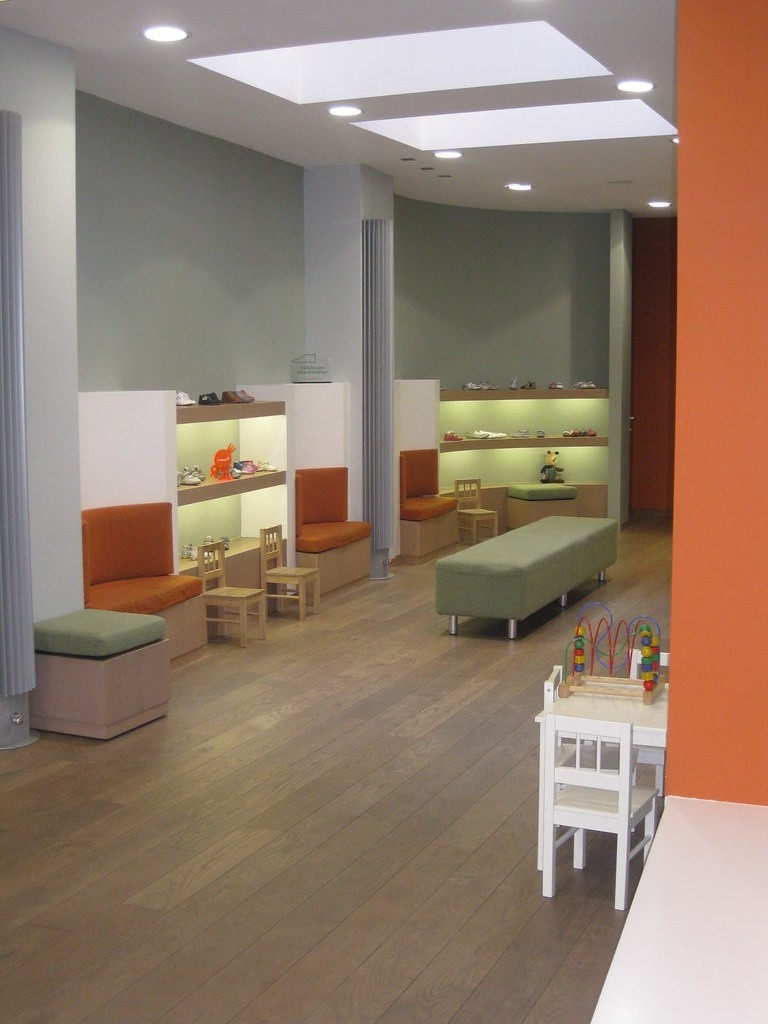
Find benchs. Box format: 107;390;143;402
435;517;619;640
397;448;458;557
293;467;374;596
78;502;207;663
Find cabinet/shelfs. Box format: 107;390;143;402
177;399;286;640
436;389;609;537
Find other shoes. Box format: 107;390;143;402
221;390;243;403
465;431;508;439
572;382;596;389
549;381;563;389
235;389;255;403
561;427;597;437
198;392;224;405
444;432;464;441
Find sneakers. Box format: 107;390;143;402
176;392;196;406
520;381;536;390
216;464;241;479
189;466;204;481
463;380;500;390
203;536;214;552
258;459;276;471
233;460;255;474
509;376;518;389
219;537;229;549
244;460;263;472
510;430;530;438
190;546;212;561
182;544;193;558
536;429;545;439
177;467;201;485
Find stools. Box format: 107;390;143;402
34;611;170;740
507;485;578;529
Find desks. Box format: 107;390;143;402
534;683;669;869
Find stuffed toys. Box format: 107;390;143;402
540;451;564;481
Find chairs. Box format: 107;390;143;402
454;478;499;546
544;665;640;832
629;648;670;798
197;540;267;648
543;714;659;911
261;525;320;620
